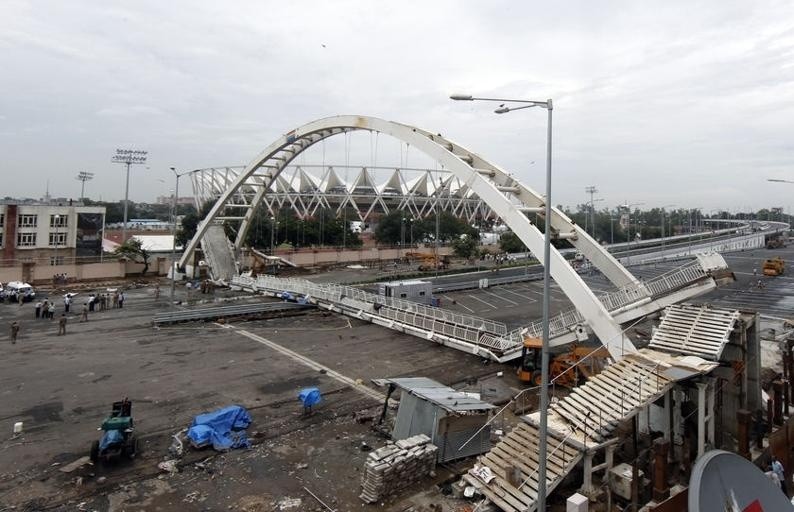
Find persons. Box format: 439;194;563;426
771;456;790;499
154;286;161;298
0;272;124;322
764;466;782;490
57;314;67;336
12;322;19;344
486;250;510;265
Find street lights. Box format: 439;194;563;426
113;148;149;249
584;187;599;238
584;199;605;231
449;93;555;512
767;179;794;185
76;170;94;203
169;166;202;311
659;203;757;258
269;217;276;256
410;218;413;265
610;204;629;252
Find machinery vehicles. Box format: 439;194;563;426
517;329;609;393
767;236;787;249
763;256;785;276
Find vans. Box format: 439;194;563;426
7;281;36;302
